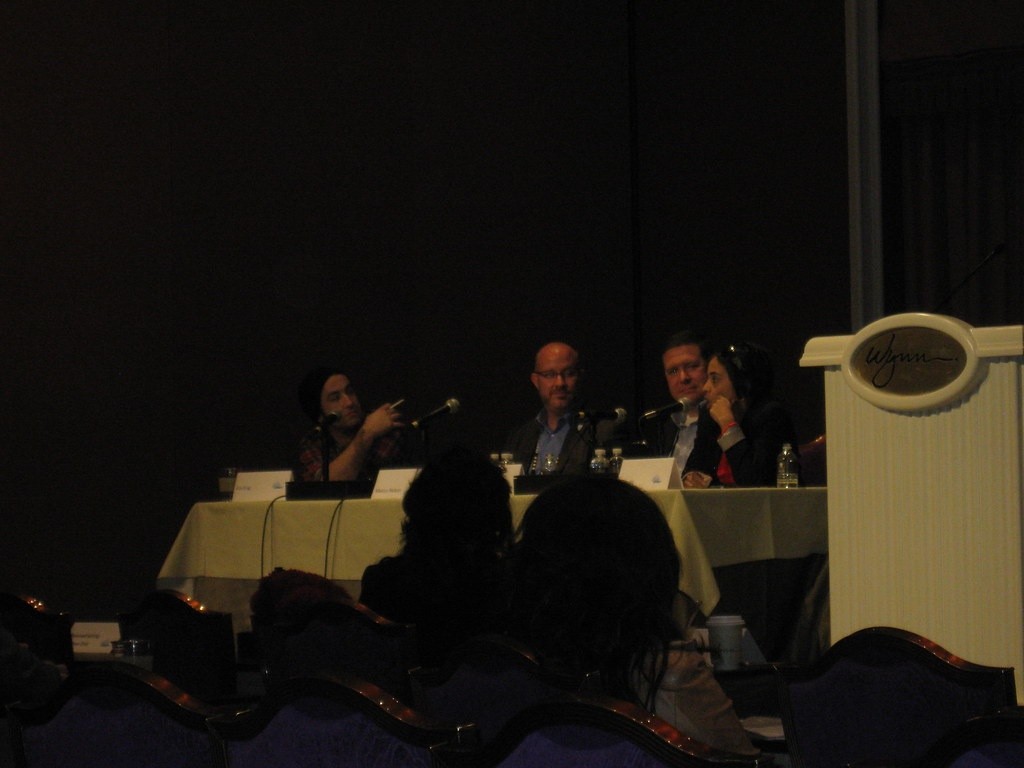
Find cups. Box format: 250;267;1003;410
216;468;236;502
705;615;745;666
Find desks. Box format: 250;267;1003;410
156;487;828;631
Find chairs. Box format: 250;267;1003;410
0;589;1024;768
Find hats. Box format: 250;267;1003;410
298;365;353;423
715;339;771;400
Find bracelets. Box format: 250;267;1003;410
722;422;736;433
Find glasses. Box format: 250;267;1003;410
536;369;575;380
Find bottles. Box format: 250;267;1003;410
776;444;798;488
591;449;609;475
542;454;557;475
490;453;515;469
609;448;624;475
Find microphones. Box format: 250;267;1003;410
311;411;344;437
574;407;627;422
642;396;693;420
409;398;460;430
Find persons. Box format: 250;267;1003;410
504;342;616;471
680;341;804;489
519;478;758;757
0;625;68;706
357;448;518;664
293;368;405;481
638;330;721;474
